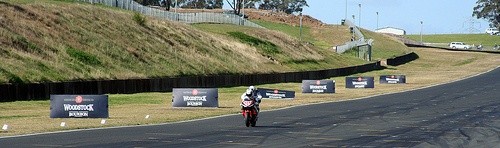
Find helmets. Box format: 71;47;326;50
246;89;254;96
249;86;256;91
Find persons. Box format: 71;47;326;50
241;86;262;121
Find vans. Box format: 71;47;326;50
486;28;499;36
450;42;471;50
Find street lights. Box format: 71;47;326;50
358;3;361;31
420;21;424;46
376;11;379;34
352;15;356;42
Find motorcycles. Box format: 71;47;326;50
240;99;260;127
